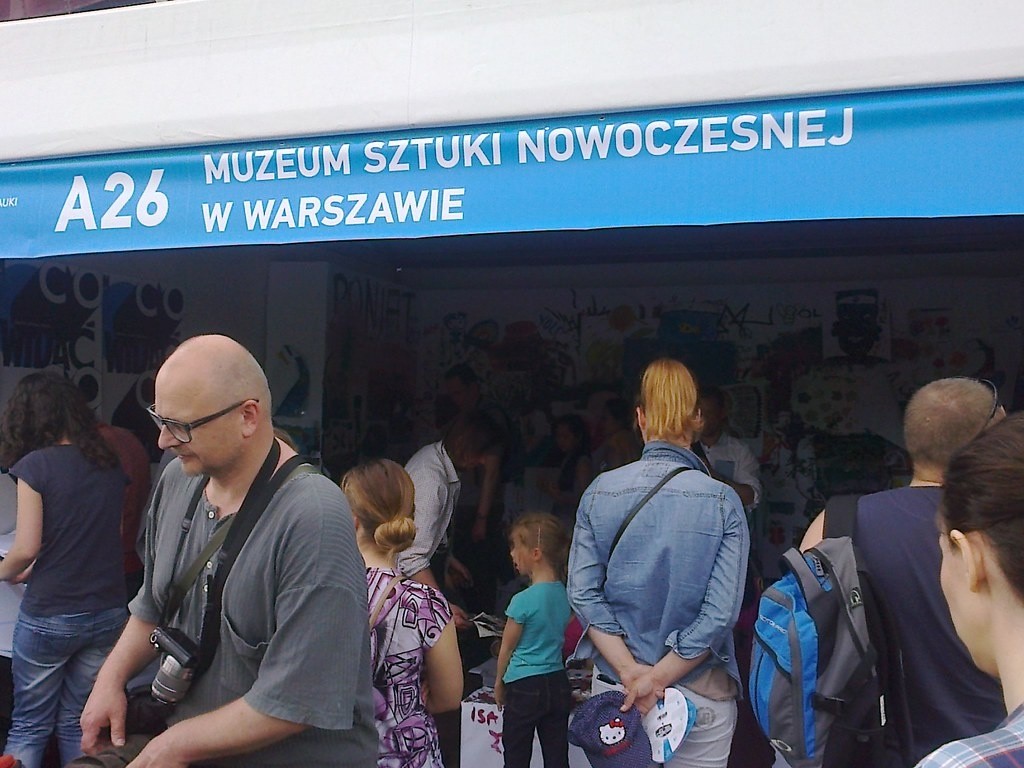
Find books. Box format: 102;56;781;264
468;611;505;638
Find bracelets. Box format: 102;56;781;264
479;513;489;521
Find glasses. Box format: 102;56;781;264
955;375;1001;433
146;399;259;443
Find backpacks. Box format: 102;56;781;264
748;494;915;768
368;576;408;719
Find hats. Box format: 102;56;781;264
567;690;653;768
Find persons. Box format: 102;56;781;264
319;330;886;695
494;513;571;768
911;410;1024;768
0;358;154;768
76;334;379;768
339;457;463;768
797;375;1010;768
561;359;750;768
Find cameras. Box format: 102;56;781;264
148;626;202;704
